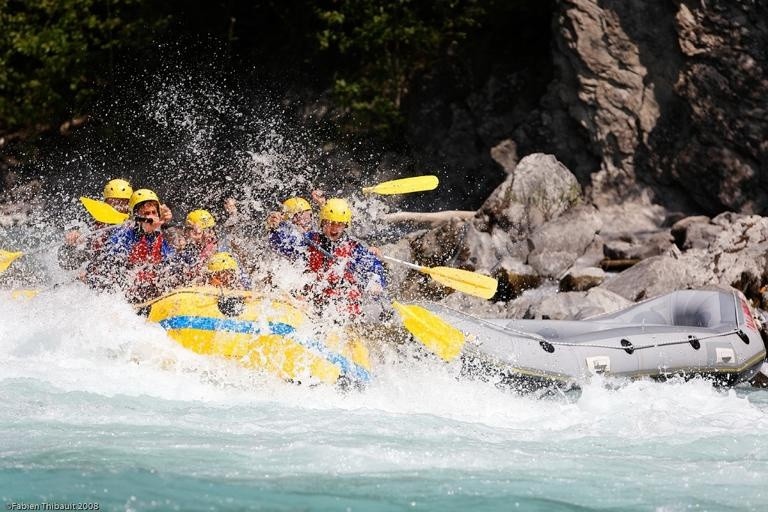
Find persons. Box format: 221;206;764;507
56;179;387;340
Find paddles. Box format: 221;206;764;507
0;225;125;275
305;235;466;359
78;197;193;229
367;252;498;299
262;175;440;201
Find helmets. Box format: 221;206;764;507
104;179;134;202
319;200;351;228
208;253;237;271
129;188;161;219
282;198;312;221
185;209;215;230
